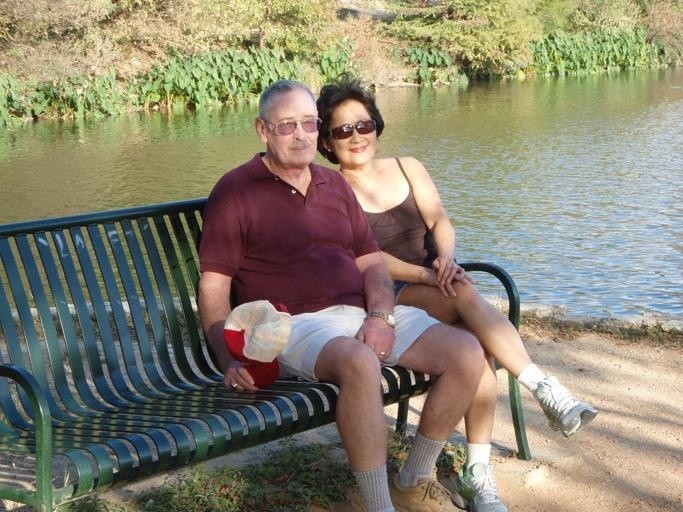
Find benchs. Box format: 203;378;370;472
0;198;535;511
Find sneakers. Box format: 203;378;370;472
535;376;598;436
390;471;460;511
450;463;506;511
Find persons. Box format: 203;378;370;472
197;81;485;512
315;70;598;512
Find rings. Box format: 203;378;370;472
230;383;238;391
380;351;390;357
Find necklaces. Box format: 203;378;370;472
266;157;309;191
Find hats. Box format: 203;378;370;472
223;299;291;388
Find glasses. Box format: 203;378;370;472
329;120;376;139
261;116;321;136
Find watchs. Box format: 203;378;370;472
365;312;396;329
